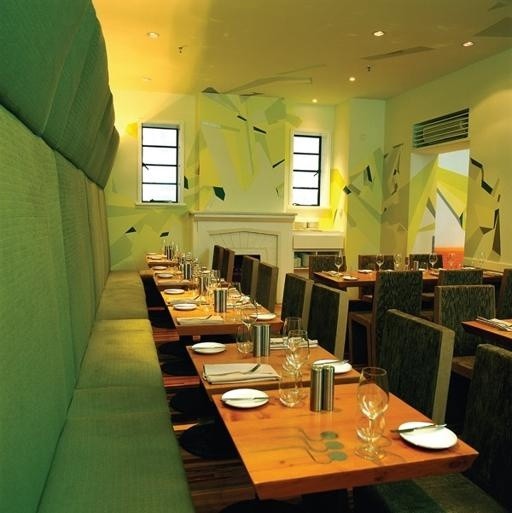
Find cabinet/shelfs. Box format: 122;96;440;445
292;231;345;269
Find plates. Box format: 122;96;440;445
249;311;276;320
146;251;196;310
312;358;352;374
398;419;458;449
344;266;476;281
222;387;268;408
191;341;226;353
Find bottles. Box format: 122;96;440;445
310;364;336;411
164;244;228;312
253;323;271;358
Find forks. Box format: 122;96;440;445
207;363;261;376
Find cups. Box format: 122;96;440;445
355;405;387;441
236;325;256;352
279;370;304;405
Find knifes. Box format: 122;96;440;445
188;345;228;349
220;396;269;401
391;422;448;433
309;358;348;364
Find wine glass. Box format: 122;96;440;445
240;298;259;357
333;250;488;276
200;266;242;321
281;317;311;397
355;366;391;461
